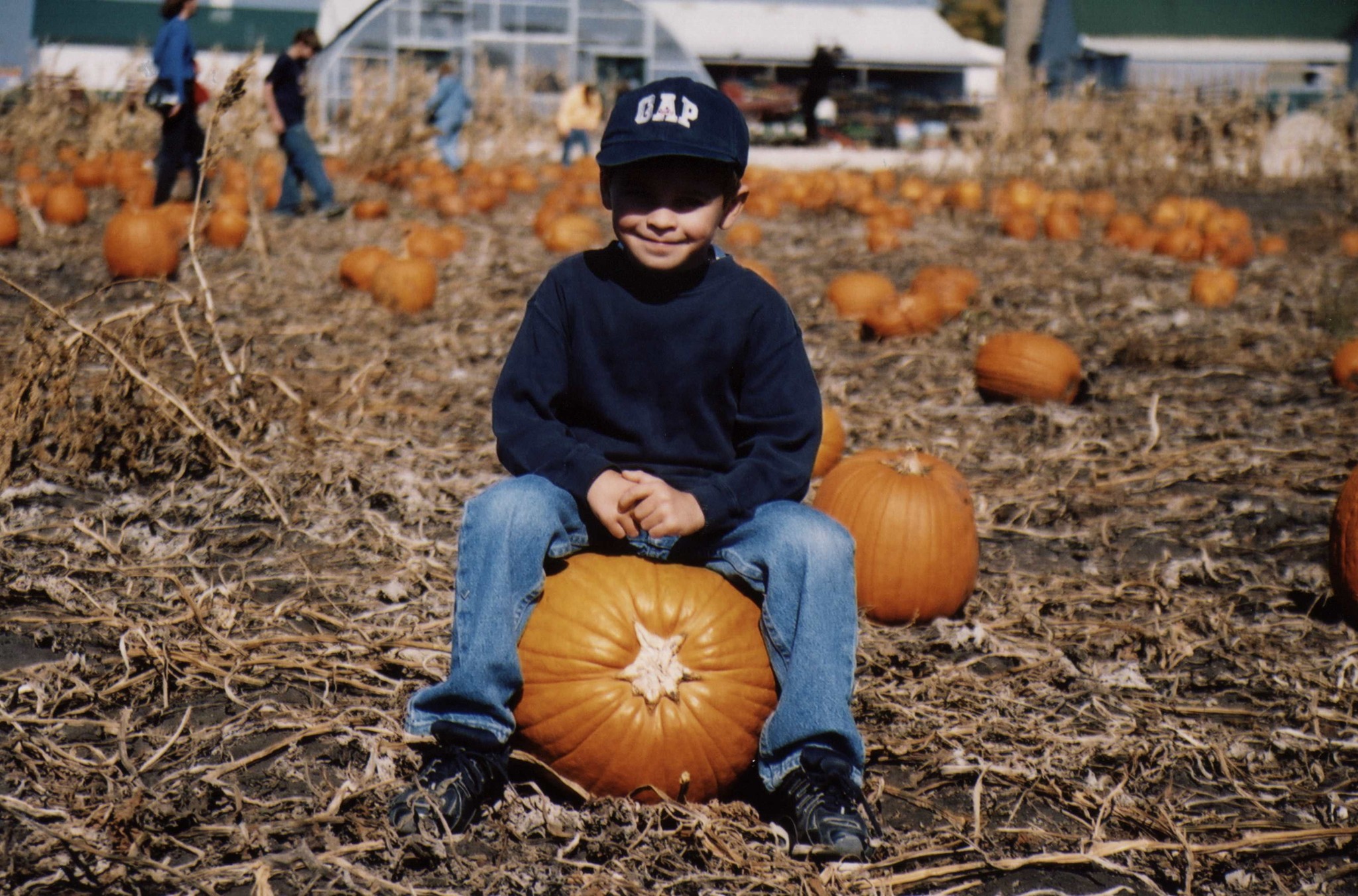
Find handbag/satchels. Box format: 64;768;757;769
425;114;440;125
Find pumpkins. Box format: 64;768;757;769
1329;463;1358;631
509;550;780;804
811;446;977;625
808;407;845;479
0;140;1358;406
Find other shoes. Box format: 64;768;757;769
312;202;351;221
271;204;306;221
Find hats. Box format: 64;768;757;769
293;29;324;52
595;77;749;178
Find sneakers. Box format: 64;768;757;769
388;720;508;836
759;745;883;862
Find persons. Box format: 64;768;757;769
390;77;880;858
799;46;844;142
424;64;474;172
555;82;603;164
154;0;209;203
265;29;345;218
